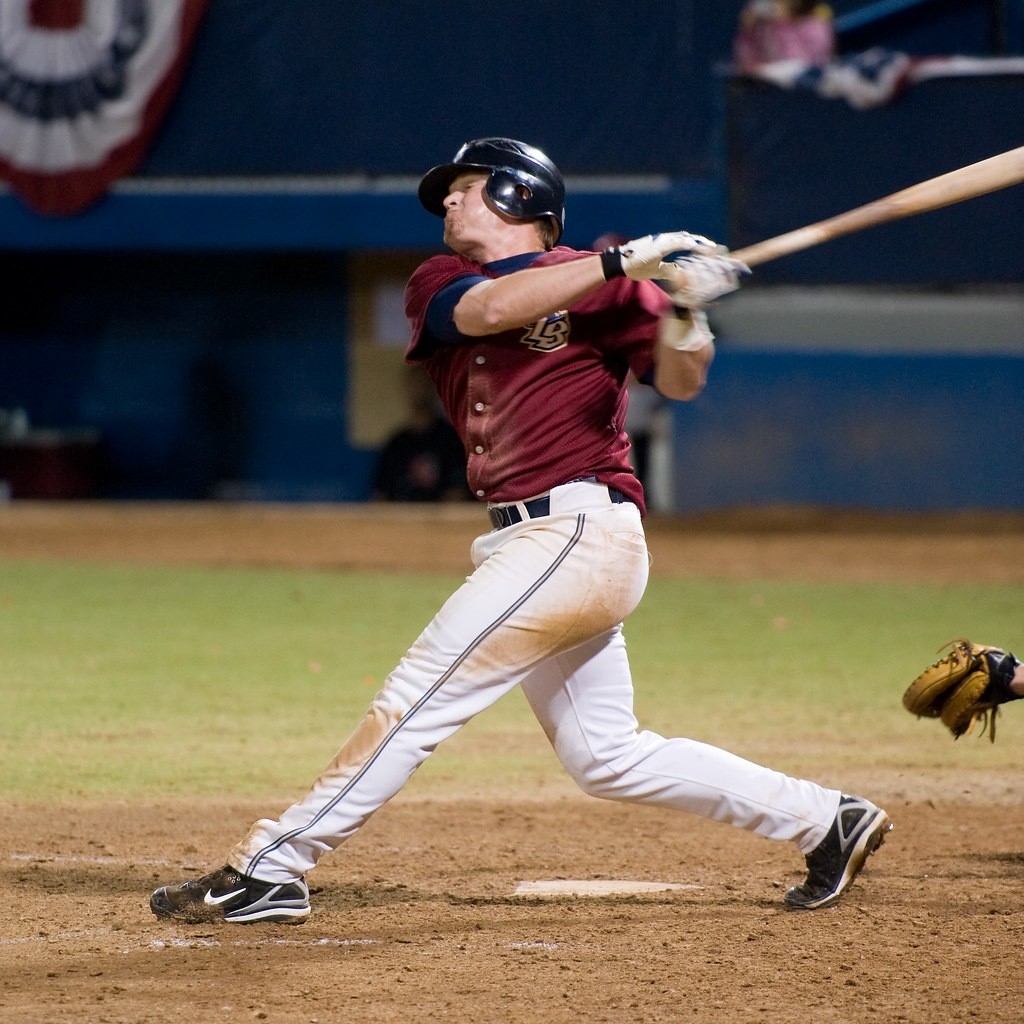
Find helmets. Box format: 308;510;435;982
418;137;566;245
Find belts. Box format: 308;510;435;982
488;484;632;532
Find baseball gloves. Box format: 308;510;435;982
902;636;1024;746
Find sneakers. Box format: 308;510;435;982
785;793;893;909
150;863;311;924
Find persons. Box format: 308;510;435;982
738;1;915;110
369;365;476;501
150;137;894;925
903;638;1024;742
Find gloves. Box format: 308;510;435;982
664;254;753;317
602;231;717;277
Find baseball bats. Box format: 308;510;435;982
667;145;1024;289
